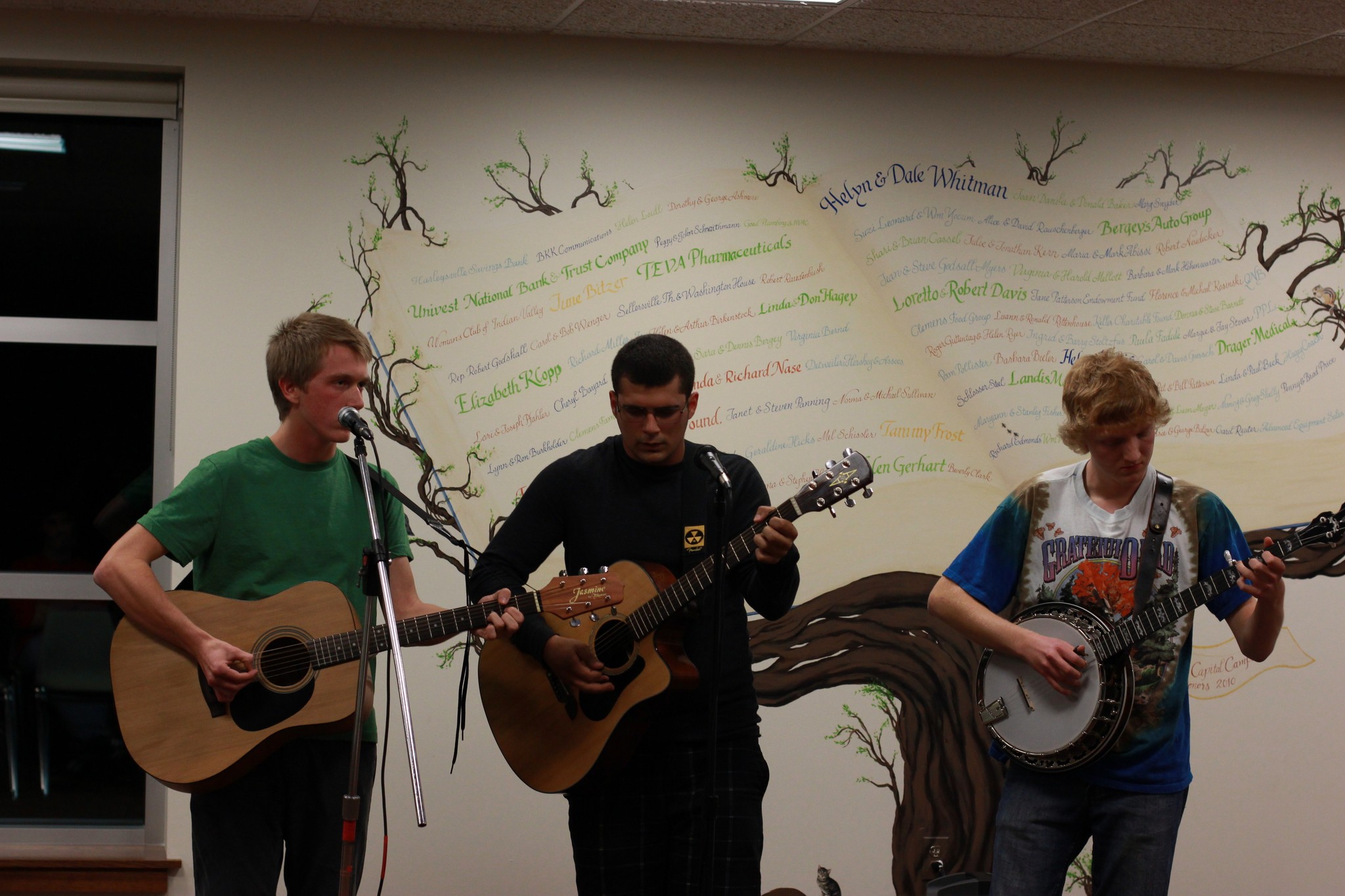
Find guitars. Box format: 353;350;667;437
971;505;1344;778
107;561;629;795
475;447;880;795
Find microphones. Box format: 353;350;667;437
338;406;374;441
693;444;732;490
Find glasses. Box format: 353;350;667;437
614;397;687;423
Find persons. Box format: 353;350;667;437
93;312;524;896
927;348;1286;896
470;334;800;896
